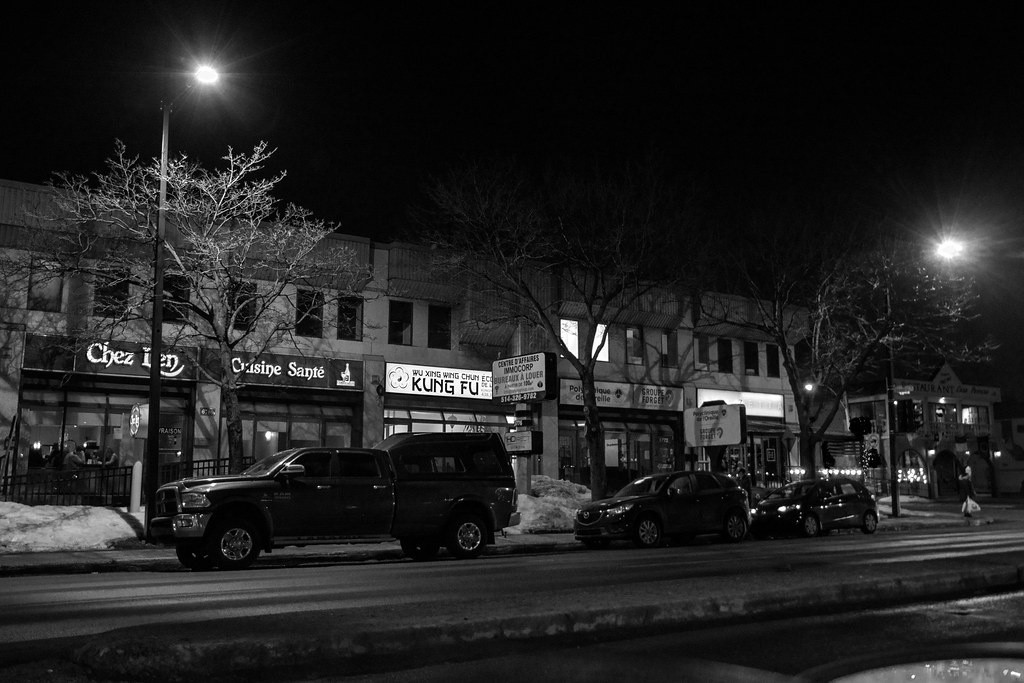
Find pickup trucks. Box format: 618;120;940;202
147;430;523;572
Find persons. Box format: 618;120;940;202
958;466;977;517
735;461;760;508
28;440;118;494
710;427;723;445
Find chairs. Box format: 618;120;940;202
28;443;55;473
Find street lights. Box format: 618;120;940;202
143;53;224;545
881;229;972;517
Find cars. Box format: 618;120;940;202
750;474;881;540
573;468;752;548
